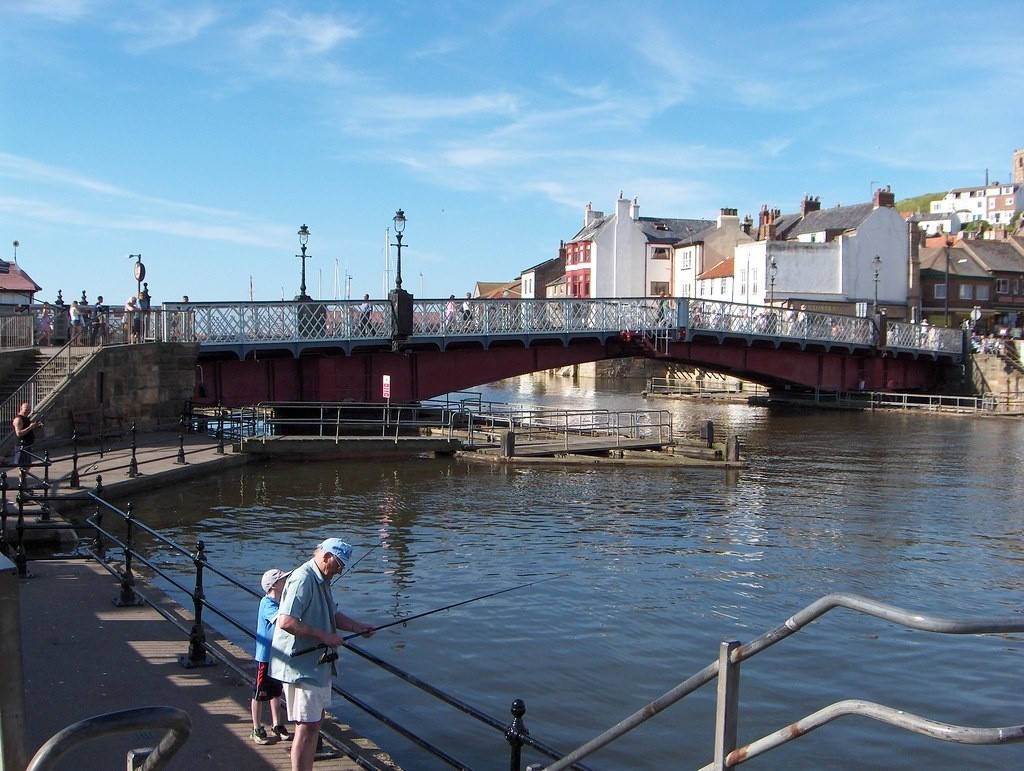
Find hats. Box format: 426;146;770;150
261;569;293;592
317;538;354;573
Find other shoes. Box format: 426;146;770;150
35;339;39;346
71;343;76;346
77;343;84;346
47;344;53;346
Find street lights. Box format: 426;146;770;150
390;208;408;293
872;255;882;314
945;241;955;325
127;254;141;298
974;306;980;327
296;225;313;301
768;257;777;313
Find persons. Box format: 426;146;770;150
463;292;473;333
360;294;376;338
35;302;53;346
267;538;377;771
63;301;86;347
649;291;673;328
446;295;457;326
181;296;188;302
691;304;1001;355
250;569;293;745
122;292;150;345
12;403;38;483
90;296;107;347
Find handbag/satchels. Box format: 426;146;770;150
79;315;85;327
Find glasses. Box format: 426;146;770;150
332;554;344;573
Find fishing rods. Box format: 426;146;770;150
81;421;135;475
34;397;65;423
331;515;416;587
291;572;575;658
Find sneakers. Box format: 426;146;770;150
270;725;292;740
249;726;270;744
